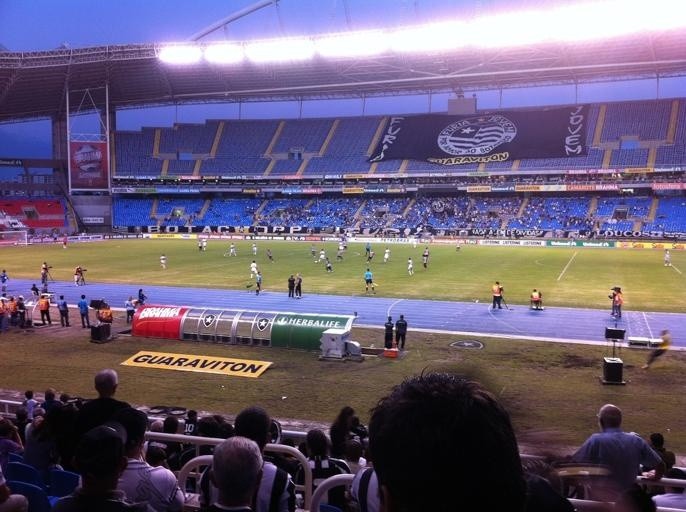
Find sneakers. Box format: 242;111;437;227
493;303;652;371
1;284;131;327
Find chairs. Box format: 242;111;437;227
112;178;401;187
1;450;80;512
406;171;685;185
144;404;356;512
359;196;510;235
0;179;78;235
116;116;383;175
374;149;604;174
111;197;363;232
586;97;684;168
509;194;685;234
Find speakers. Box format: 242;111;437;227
91;324;111;340
603;357;623;382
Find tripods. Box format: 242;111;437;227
44;271;54;284
501;295;508;309
77;273;85;286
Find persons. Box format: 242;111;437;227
384;315;393;348
0;259;148;328
641;330;673;369
201;237;207;252
614;289;624;318
491;281;504;309
223;237;432;300
664;249;672;267
158;254;167;271
530;289;543;308
395;314;407;348
196;239;201;250
61;237;67;249
609;287;618;316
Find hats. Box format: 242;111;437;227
75;421;128;468
